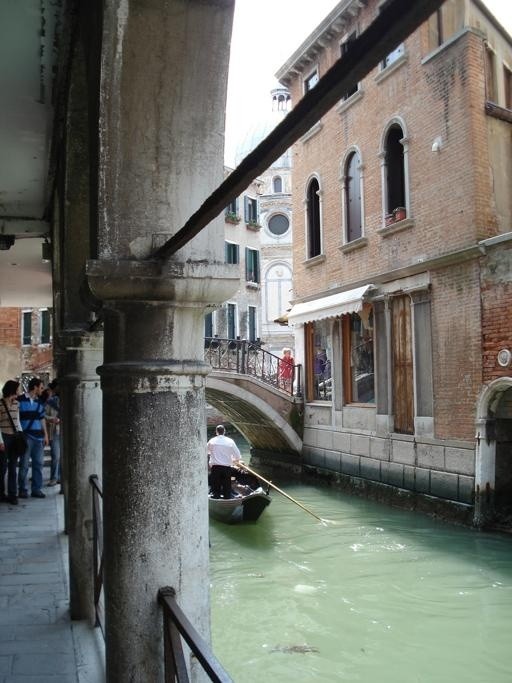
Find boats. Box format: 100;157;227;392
208;453;272;526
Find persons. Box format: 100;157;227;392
206;424;242;498
315;339;326;381
279;347;294;392
0;375;60;505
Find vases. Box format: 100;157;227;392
384;212;395;227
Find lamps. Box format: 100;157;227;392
0;233;15;251
40;236;53;263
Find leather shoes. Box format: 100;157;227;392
0;490;45;504
47;480;56;485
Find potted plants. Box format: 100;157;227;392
393;206;406;221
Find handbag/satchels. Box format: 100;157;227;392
5;430;27;457
